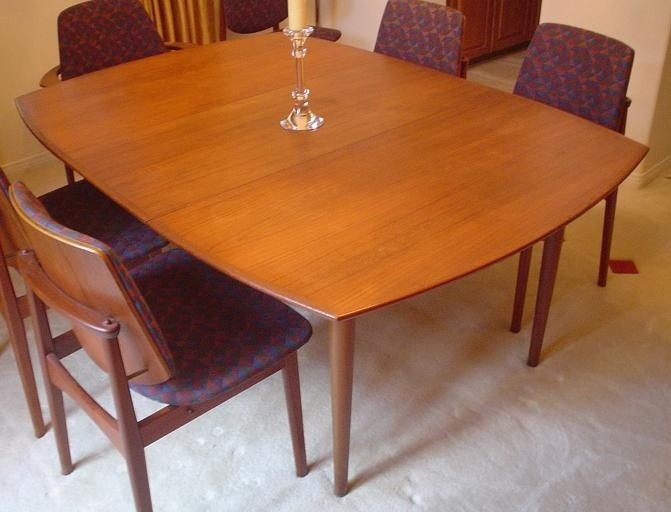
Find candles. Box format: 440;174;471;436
288;0;317;30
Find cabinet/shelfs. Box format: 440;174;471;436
447;0;542;65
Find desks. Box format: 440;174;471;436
15;31;652;499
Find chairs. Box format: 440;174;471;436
508;21;637;370
37;1;202;184
6;179;317;511
219;0;342;45
370;1;468;81
0;166;174;441
